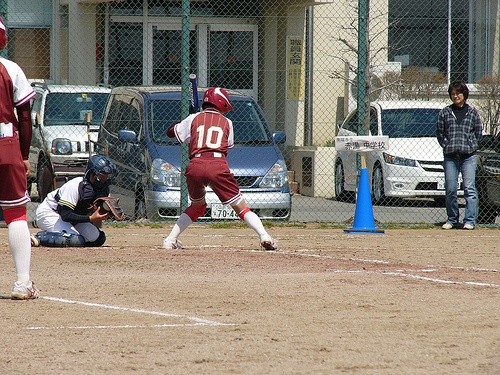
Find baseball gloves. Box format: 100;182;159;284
90;196;124;223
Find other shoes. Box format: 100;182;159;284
31;236;40;246
441;223;453;229
463;224;473;230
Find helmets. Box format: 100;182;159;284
202;87;234;112
84;155;114;179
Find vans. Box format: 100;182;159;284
87;86;291;222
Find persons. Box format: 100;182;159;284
435;80;483;230
0;16;41;300
162;86;278;251
30;154;120;248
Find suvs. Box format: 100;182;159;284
26;82;117;204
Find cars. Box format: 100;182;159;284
477;131;500;225
334;100;466;207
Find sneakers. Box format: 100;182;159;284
10;281;40;299
162;239;182;249
260;235;277;250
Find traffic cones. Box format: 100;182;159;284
342;168;384;234
